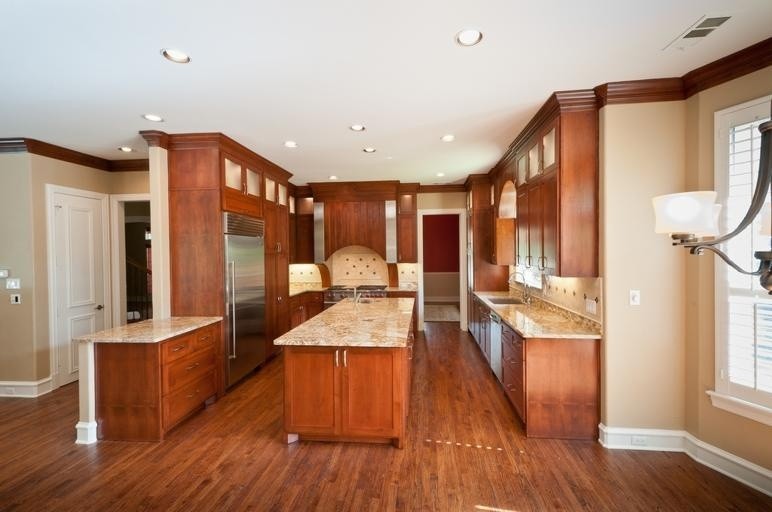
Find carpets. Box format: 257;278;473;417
425;304;460;322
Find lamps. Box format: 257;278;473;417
652;121;772;295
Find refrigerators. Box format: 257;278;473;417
223;211;266;388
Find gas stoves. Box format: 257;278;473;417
325;284;386;301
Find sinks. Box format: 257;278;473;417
489;297;523;305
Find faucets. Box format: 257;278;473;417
507;271;528;304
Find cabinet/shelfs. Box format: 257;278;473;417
306;291;324;319
464;175;510;331
220;152;263;218
490;180;516;265
387;292;418;339
500;323;526;425
94;317;225;443
283;345;402;440
264;202;290;358
514;112;600;277
479;313;491;365
290;292;305;330
468;301;482;345
263;172;289;210
